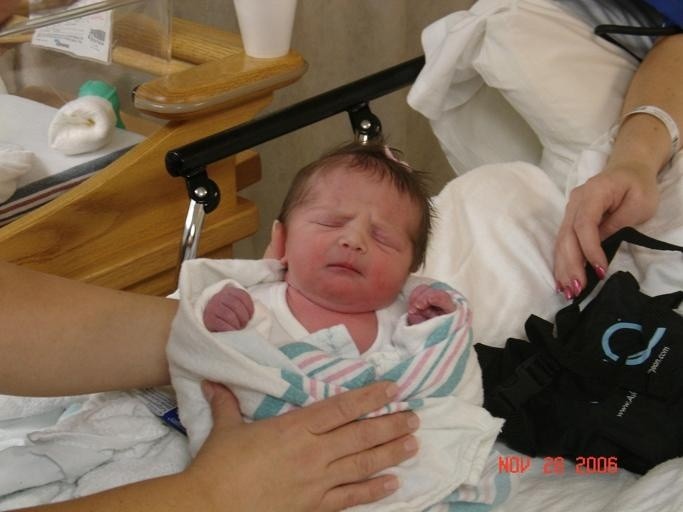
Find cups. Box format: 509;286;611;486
232;1;300;60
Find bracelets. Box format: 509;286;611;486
608;105;681;157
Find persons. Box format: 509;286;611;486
0;258;419;512
554;33;683;300
203;132;457;360
